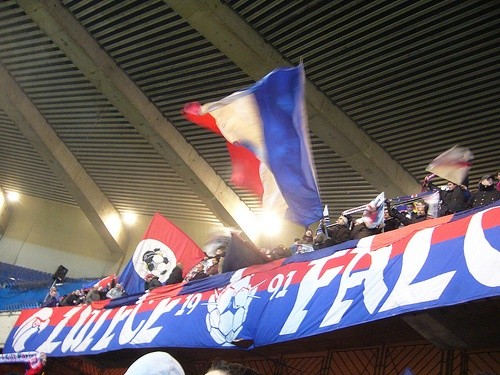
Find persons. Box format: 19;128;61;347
389;203;428;225
425;179;472;215
403;203;416;214
378;207;399;231
471;175;500;206
322;215;352;245
168;263;184;286
191;264;209;281
44;286;128;308
291;238;303;249
209;255;220;275
144;273;162;288
260;229;324;259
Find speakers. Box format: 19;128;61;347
51;265;68;282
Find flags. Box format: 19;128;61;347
118;211;207;296
182;59;325;227
426;144;475;186
